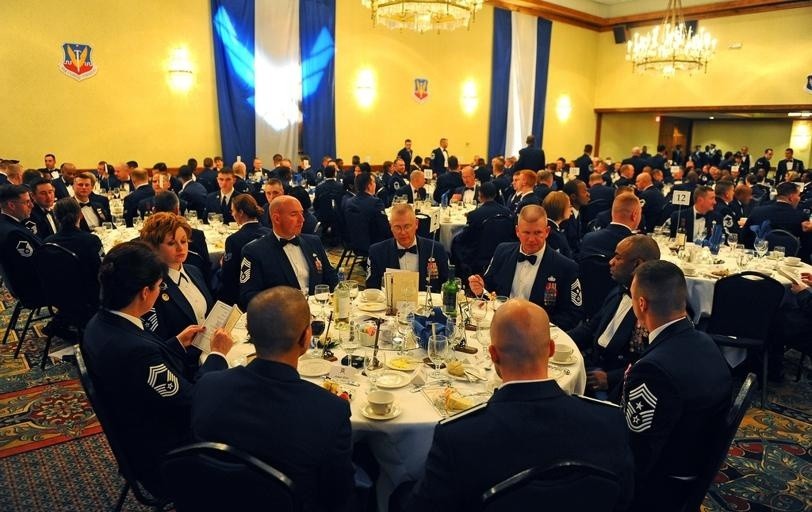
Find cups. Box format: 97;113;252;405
786;256;801;264
367;391;395;416
552;344;573;362
683;266;696;275
360;288;380;300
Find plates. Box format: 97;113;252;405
227;289;582;422
642;233;805;280
90;221;241;254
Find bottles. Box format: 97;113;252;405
107;187;112;200
675;218;687;250
440;265;459;318
392;337;404;356
119;184;126;200
332;268;351;330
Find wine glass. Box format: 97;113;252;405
100;210;224;239
231;280;510;395
391;193;478;222
646;225;787;281
114;188;120;199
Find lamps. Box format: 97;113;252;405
627;2;715;79
364;0;484;30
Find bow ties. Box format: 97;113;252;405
518;253;537;265
44;208;53;216
696;213;706;219
280;238;298;247
397;245;417;258
464;186;472;190
619;287;632;298
79;203;91;208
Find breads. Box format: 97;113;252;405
391;358;410;368
446;395;473;411
322;379;339;392
113;240;122;245
448;361;465;376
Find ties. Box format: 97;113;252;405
222;196;226;207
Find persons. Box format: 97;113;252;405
607;260;738;511
388;301;635;512
81;242;226;502
0;134;812;350
189;284;360;511
565;234;661;401
